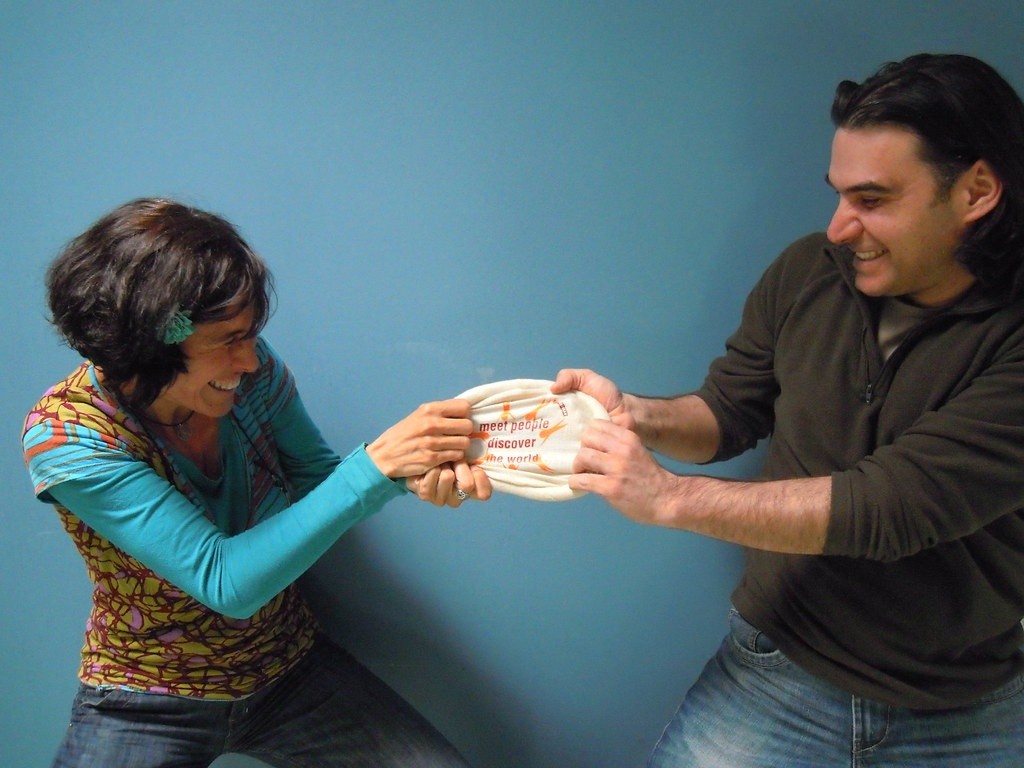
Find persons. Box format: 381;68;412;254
550;48;1024;768
21;193;491;768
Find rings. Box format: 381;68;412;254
452;489;470;501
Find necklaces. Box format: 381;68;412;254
139;409;291;526
